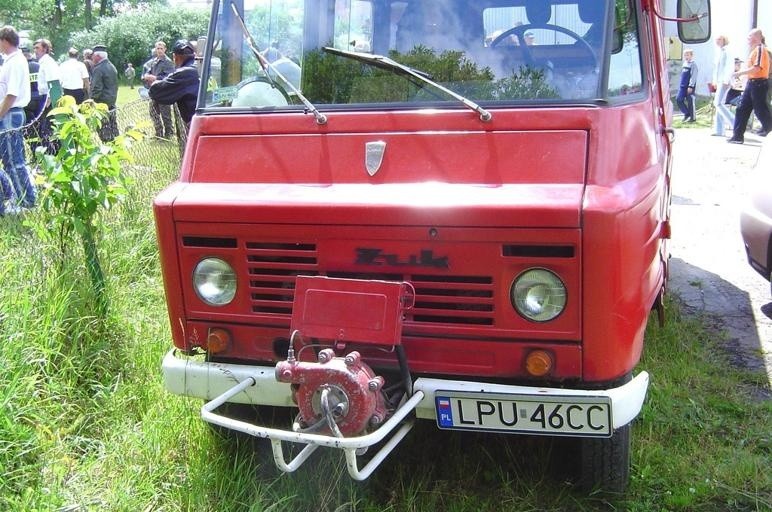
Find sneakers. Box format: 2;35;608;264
726;137;744;145
2;198;38;215
756;123;771;137
682;113;696;124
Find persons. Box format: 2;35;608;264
484;22;536;46
711;35;735;135
725;58;754;130
1;25;36;214
0;40;198;171
761;36;771;120
261;40;283;67
675;49;698;124
728;28;771;144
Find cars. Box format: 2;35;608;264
740;130;771;321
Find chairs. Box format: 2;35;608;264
707;82;733;130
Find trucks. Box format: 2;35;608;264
150;1;713;492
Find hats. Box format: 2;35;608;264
90;45;106;55
169;39;197;52
